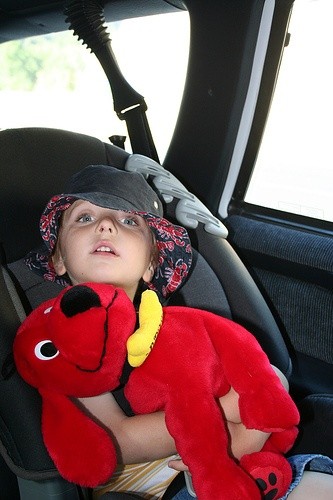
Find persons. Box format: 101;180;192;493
27;166;333;500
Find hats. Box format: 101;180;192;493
23;165;193;306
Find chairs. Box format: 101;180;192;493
0;128;333;500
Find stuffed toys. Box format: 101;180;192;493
15;281;300;500
